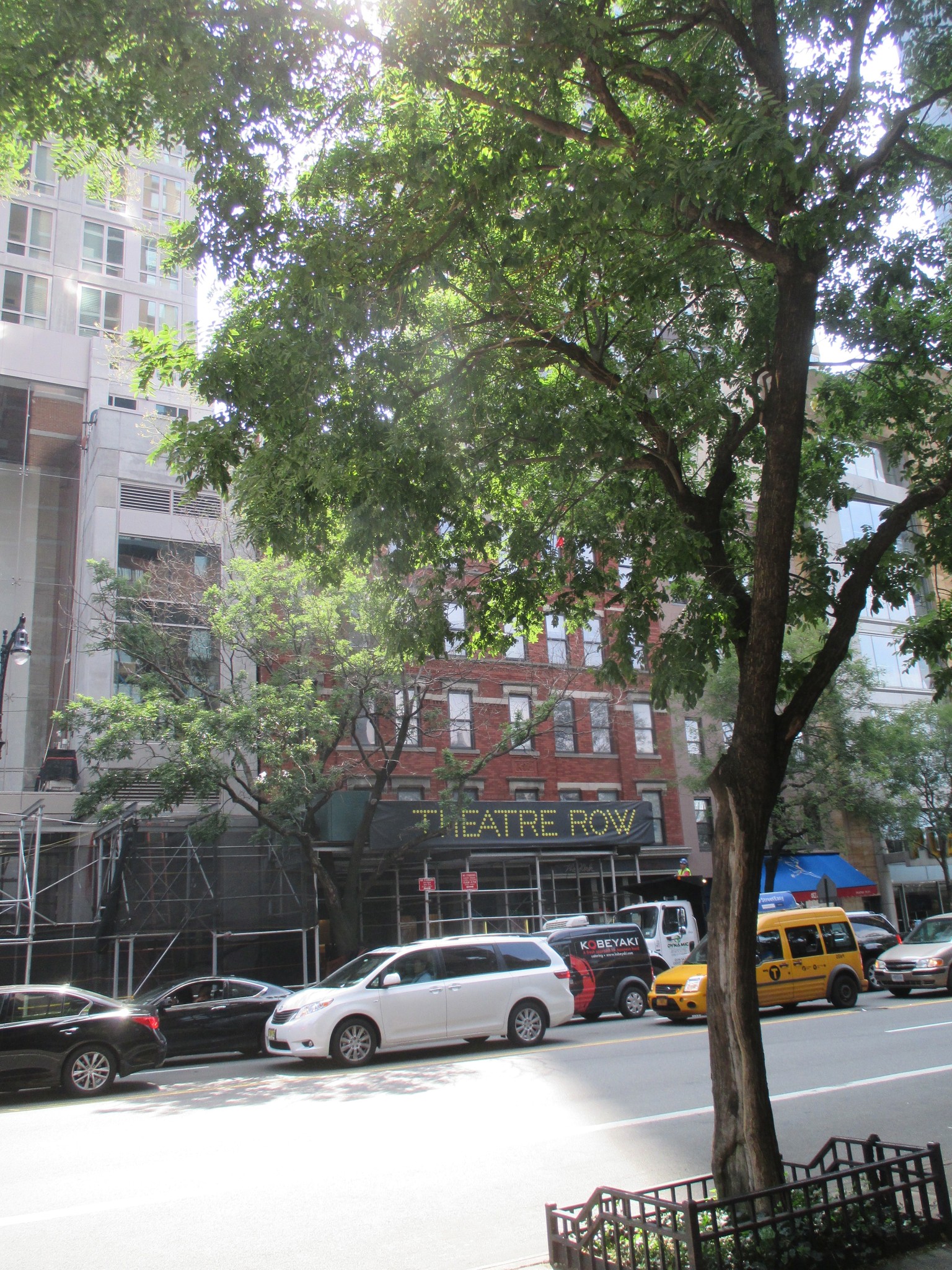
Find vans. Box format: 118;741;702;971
528;915;655;1022
647;906;869;1025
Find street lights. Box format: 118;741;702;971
2;610;32;759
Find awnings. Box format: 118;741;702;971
759;854;878;903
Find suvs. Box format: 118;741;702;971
830;910;902;993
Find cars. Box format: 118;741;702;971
874;912;952;997
264;932;575;1067
106;992;296;1057
0;984;169;1098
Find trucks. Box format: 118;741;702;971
605;875;801;978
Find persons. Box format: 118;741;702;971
167;984;212;1006
411;957;432;984
673;859;692;876
756;939;775;962
